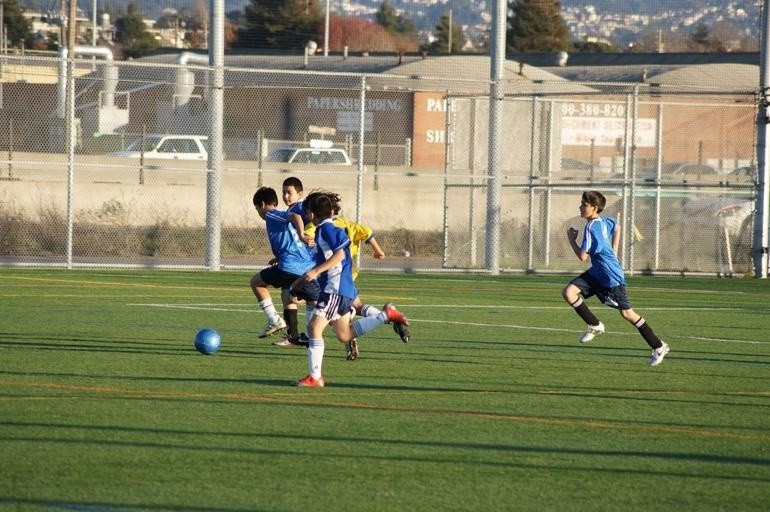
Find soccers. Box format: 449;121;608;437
195;328;221;354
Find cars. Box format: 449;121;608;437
560;155;598;172
649;162;721;176
264;138;353;168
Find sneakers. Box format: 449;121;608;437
258;318;286;338
272;334;293;345
580;321;605;343
288;332;309;347
383;303;410;343
291;374;324;386
345;338;358;360
648;340;669;366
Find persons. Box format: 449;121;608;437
250;185;316;340
270;177;316;347
301;188;410;361
562;191;670;365
293;194;410;387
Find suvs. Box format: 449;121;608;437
109;133;226;162
677;166;770;266
